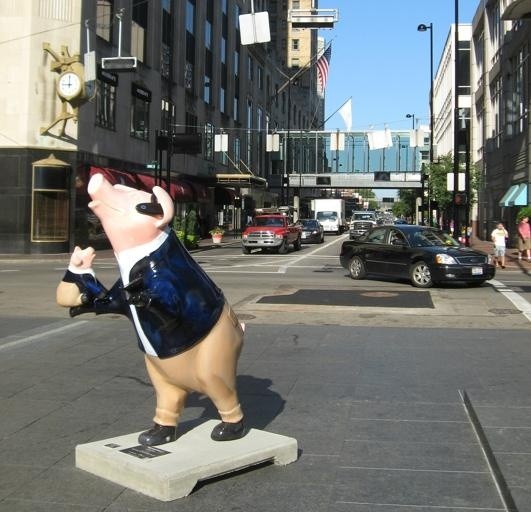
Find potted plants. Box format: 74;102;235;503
208;225;225;244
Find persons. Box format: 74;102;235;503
490;223;509;268
449;219;472;246
517;216;531;263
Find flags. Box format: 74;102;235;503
315;44;331;94
338;99;352;134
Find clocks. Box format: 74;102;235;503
58;72;81;99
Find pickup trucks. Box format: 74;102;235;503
241;215;305;255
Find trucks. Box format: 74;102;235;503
314;199;347;236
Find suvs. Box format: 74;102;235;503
346;210;383;241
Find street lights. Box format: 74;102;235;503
418;22;438;227
407;114;416;130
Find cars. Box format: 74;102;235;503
340;224;497;288
295;219;325;244
